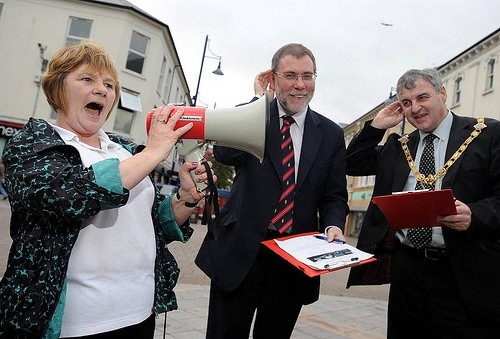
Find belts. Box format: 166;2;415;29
398;244;465;261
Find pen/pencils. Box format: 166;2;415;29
313;234;346;244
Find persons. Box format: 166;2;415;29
194;44;350;339
0;41;217;339
346;67;500;339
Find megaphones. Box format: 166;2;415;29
146;93;266;192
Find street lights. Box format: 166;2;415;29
384;86;405;137
193;34;224;106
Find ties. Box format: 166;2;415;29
270;116;296;234
406;133;435;250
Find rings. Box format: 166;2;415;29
157;119;167;124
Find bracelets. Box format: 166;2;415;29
176;185;198;207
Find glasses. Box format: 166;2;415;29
275;71;317;81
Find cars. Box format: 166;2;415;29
155;183;178;198
